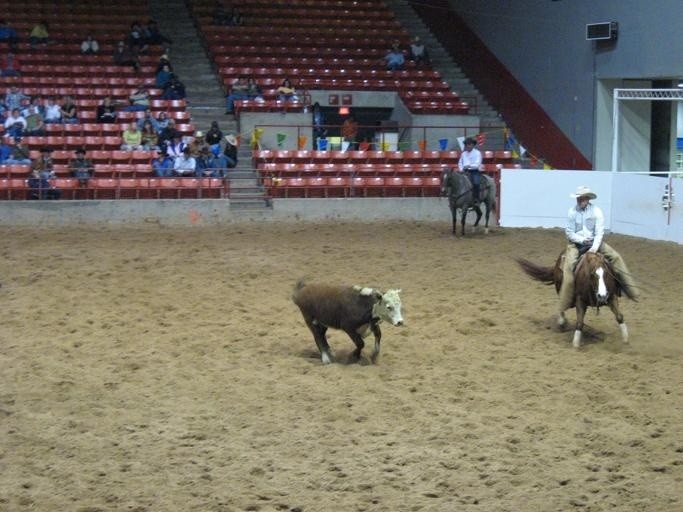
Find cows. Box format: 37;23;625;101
288;280;405;366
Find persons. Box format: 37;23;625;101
340;114;358;151
224;75;251;115
456;136;483;210
96;86;237;178
246;76;265;102
1;17;49;77
406;35;429;71
228;6;244;26
310;99;328;151
1;84;95;198
81;18;187;100
553;185;643;329
377;44;406;69
275;78;298;104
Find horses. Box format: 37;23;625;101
510;243;629;350
438;165;497;237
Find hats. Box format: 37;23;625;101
463;137;477;146
15;136;21;141
158;131;238;157
75;147;86;154
570;186;597;199
39;147;53;152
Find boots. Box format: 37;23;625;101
473;184;480;207
